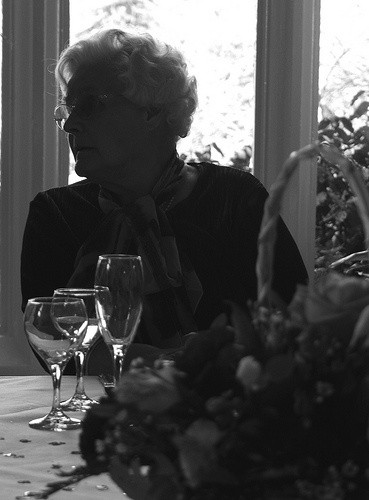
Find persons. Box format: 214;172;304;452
20;25;309;373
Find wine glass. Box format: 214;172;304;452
51;288;112;411
22;298;85;431
93;254;144;388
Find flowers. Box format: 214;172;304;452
76;272;369;499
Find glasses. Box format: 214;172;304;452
51;91;121;131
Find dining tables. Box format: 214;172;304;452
1;375;129;500
